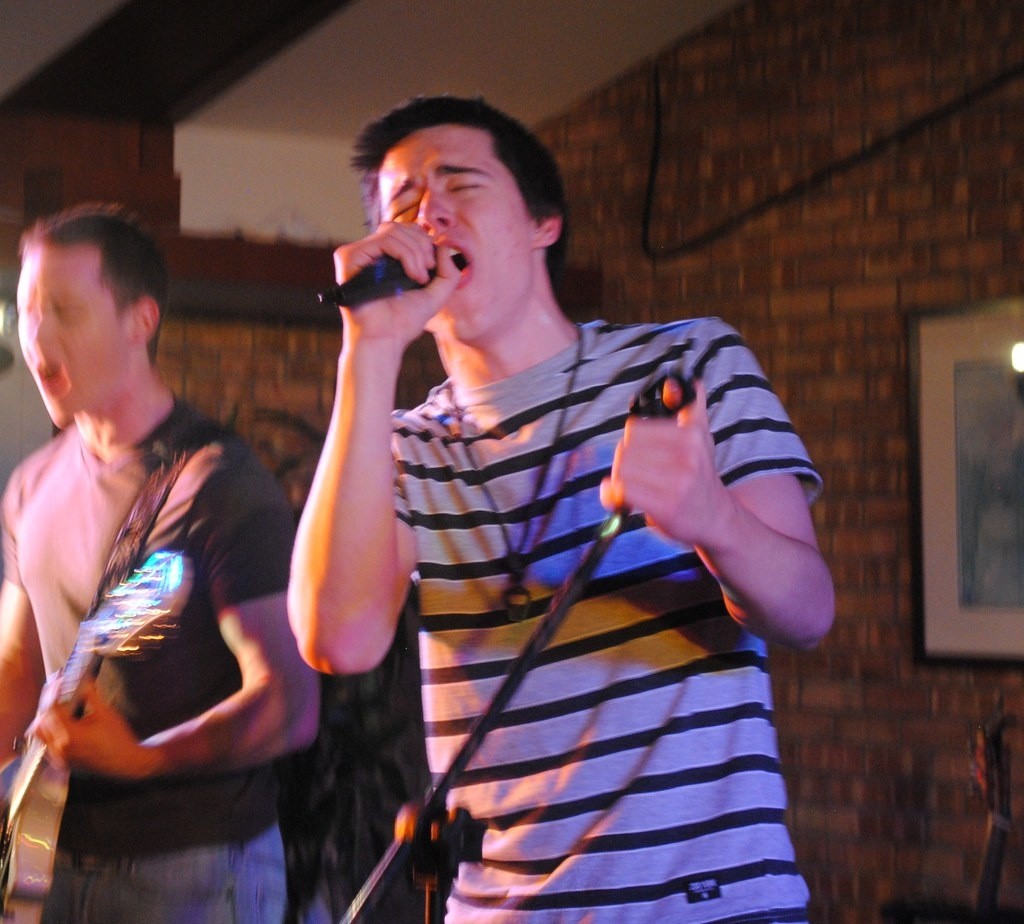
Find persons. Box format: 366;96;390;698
286;95;834;924
0;203;320;924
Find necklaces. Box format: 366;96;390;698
456;327;583;622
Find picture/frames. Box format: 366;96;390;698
903;294;1024;672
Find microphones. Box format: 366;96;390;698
317;242;437;308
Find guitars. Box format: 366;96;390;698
0;551;193;924
